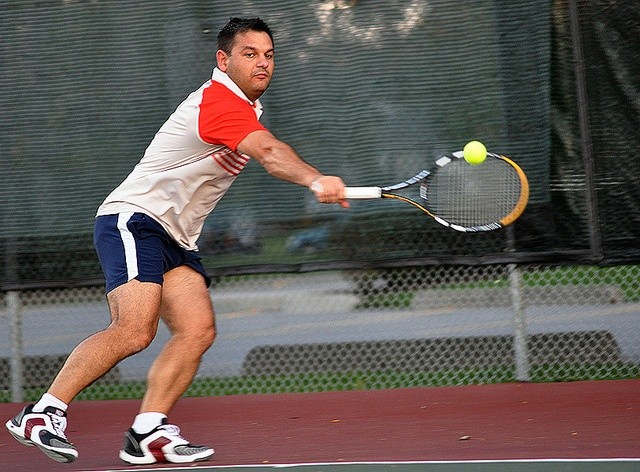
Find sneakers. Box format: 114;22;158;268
120;418;215;465
5;404;79;463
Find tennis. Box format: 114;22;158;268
464;141;487;165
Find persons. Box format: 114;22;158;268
6;17;348;463
